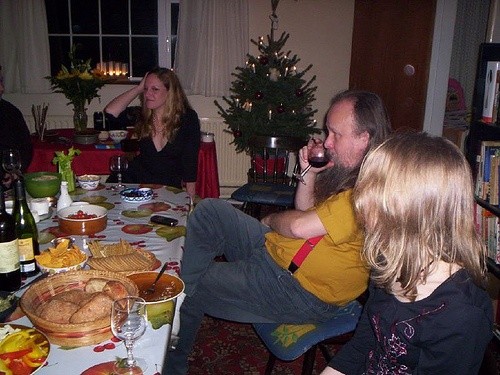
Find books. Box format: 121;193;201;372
466;60;500;267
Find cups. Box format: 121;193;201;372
2;148;22;172
5;198;88;224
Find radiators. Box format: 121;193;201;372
22;111;252;186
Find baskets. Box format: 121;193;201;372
21;271;139;347
87;240;156;273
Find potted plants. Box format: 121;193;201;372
215;30;323;176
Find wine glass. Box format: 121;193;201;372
110;296;148;375
110;155;129;191
293;143;331;184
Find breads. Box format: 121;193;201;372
35;277;130;323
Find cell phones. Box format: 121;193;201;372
150;215;178;227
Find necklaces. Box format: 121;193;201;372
396;260;435;283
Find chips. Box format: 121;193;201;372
36;239;85;268
35;240;86;268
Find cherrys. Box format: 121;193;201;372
68;210;97;219
94;336;122;352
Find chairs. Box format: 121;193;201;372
251;293;365;375
232;133;309;216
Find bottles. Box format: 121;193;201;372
57;181;72;210
195;132;220;199
0;178;41;291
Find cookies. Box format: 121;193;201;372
86;237;156;272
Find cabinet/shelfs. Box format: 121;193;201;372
467;42;500;342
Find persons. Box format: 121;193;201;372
165;91;390;374
104;68;201;202
0;79;34;190
320;132;500;375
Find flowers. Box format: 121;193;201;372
42;43;108;125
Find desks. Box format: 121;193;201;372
28;128;221;199
0;183;195;375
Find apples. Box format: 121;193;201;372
68;188;115;210
37;226;69;245
80;361;144;375
121;202;172;234
89;183;105;190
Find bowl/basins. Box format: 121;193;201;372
46;131;128;144
58;204;108;235
36;248;90;275
0;291;18;322
78;175;102;190
24;171;62;197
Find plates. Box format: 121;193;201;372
120;189;153;202
0;323;51;375
126;272;186;304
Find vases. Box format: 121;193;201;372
71;108;89;132
